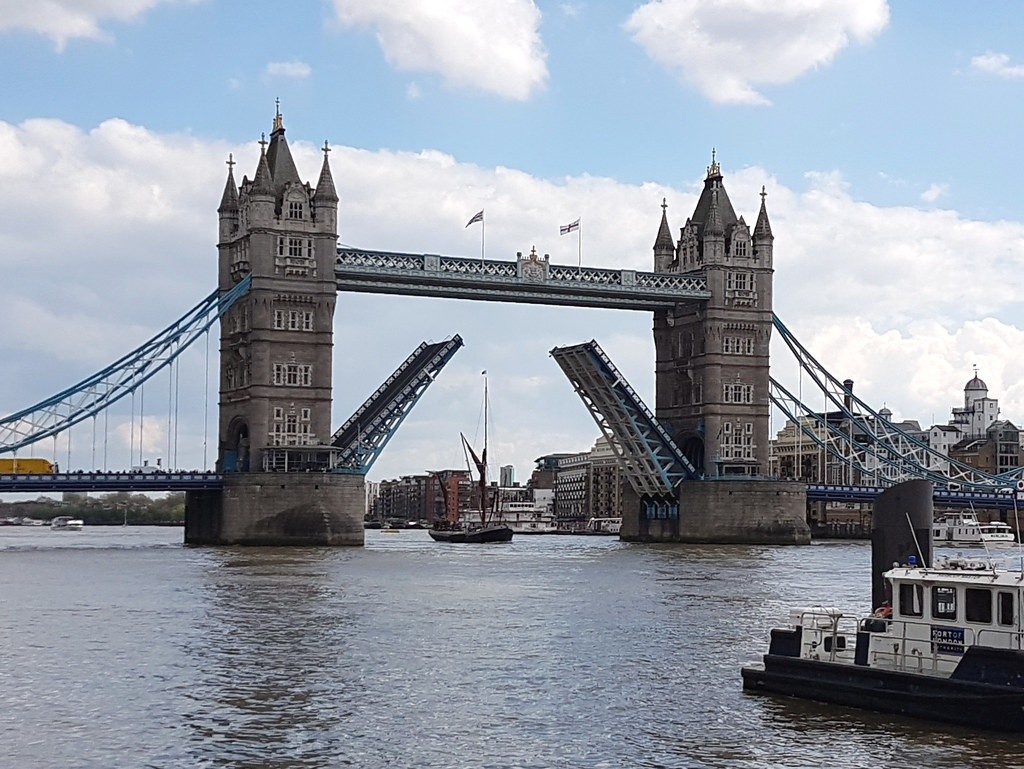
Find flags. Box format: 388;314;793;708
560;219;579;235
466;211;483;228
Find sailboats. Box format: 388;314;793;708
424;369;514;544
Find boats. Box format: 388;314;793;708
50;516;83;532
933;510;1017;549
458;488;559;535
740;498;1023;734
